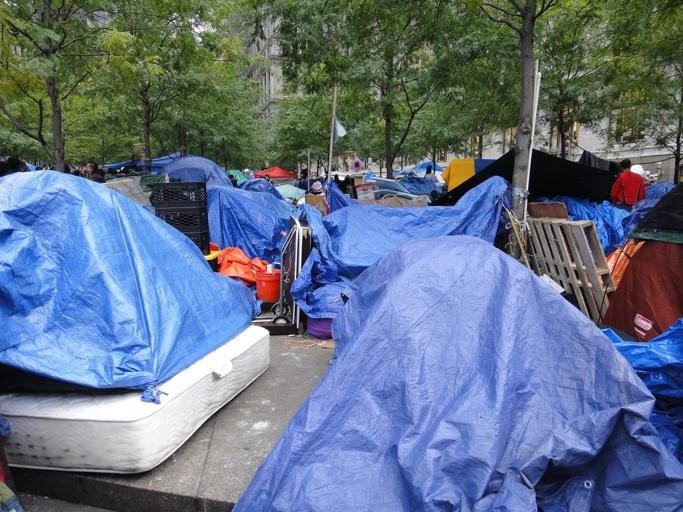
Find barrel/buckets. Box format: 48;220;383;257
255;268;281;301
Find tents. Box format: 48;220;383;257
593;181;683;342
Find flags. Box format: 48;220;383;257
331;107;347;145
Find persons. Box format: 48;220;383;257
318;170;354;197
423;165;438;183
228;174;238;188
263;172;274;187
0;155;146;184
609;158;646;207
